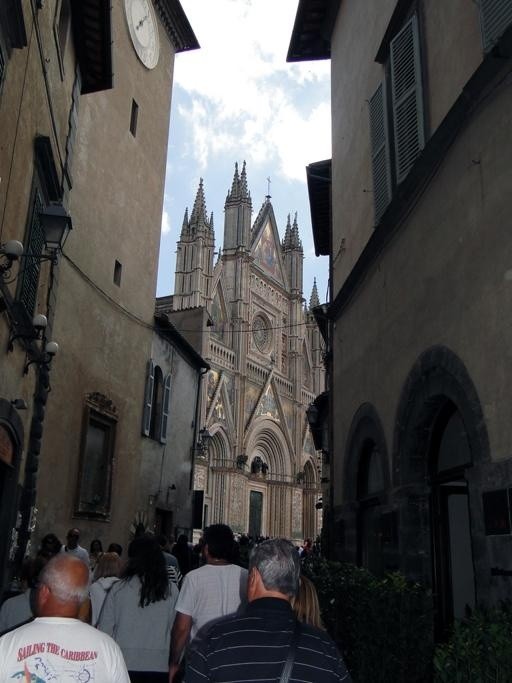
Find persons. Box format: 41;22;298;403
1;522;352;682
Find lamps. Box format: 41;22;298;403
190;427;210;456
0;194;73;375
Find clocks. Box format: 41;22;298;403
123;1;162;71
